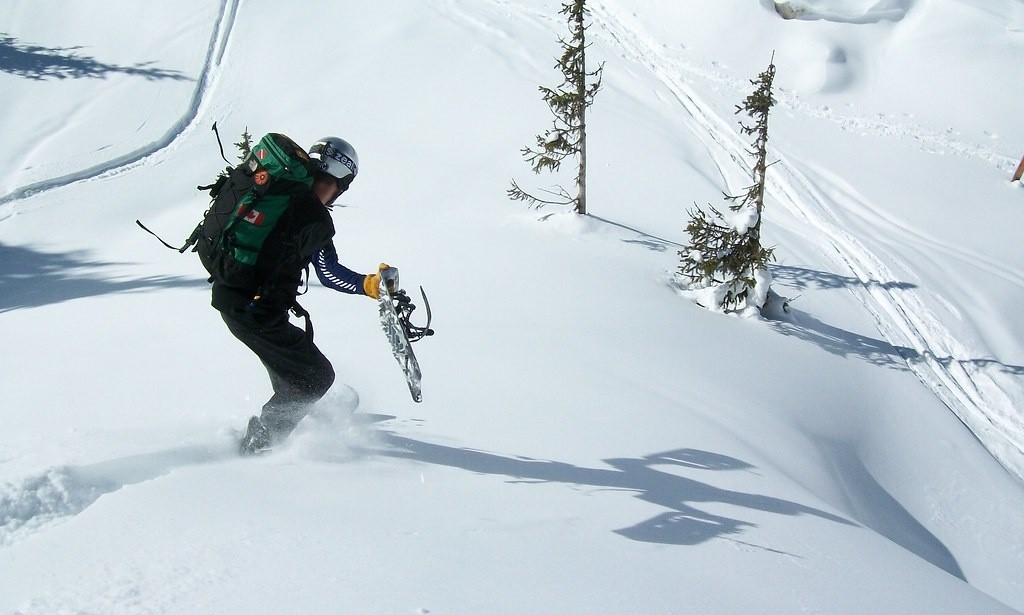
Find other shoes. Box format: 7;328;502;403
240;415;286;456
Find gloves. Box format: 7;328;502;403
364;263;396;301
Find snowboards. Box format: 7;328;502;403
298;379;360;423
375;262;425;406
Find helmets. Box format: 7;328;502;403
309;137;357;183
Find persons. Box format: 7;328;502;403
212;137;399;458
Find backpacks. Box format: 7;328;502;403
135;121;311;292
